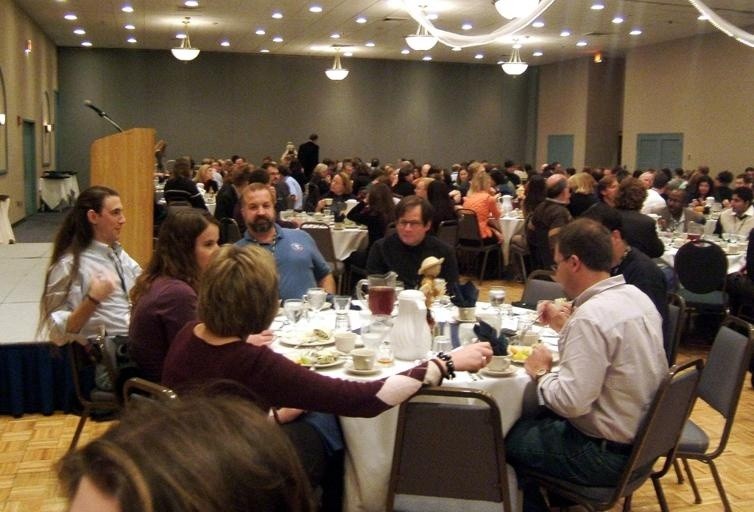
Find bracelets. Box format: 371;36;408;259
437;352;455;379
85;293;100;307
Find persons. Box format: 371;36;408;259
51;381;317;512
158;244;494;512
126;207;220;399
154;134;754;374
498;220;670;511
36;186;141;390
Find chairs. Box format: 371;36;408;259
674;240;731;330
530;356;705;512
99;336;122;406
68;342;122;450
456;209;501;286
521;269;566;306
509;242;528;282
388;386;512;512
220;217;242;244
438;221;457;246
348;222;396;296
300;221;347;296
124;377;179;415
666;292;687;367
658;316;754;511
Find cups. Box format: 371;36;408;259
487;357;511;371
325;199;333;205
356;271;398;315
516;329;541;346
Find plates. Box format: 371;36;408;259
279;336;382;376
507;344;534;363
481;364;518;376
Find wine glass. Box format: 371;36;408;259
284;281;514;356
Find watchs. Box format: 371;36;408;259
535;368;552;385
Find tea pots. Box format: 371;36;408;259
498;195;514;211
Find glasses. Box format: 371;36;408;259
399;219;419;228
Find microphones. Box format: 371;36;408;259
80;95;108;119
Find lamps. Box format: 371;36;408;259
325;45;349;80
491;0;541;20
403;5;437;51
171;16;200;63
500;38;529;79
